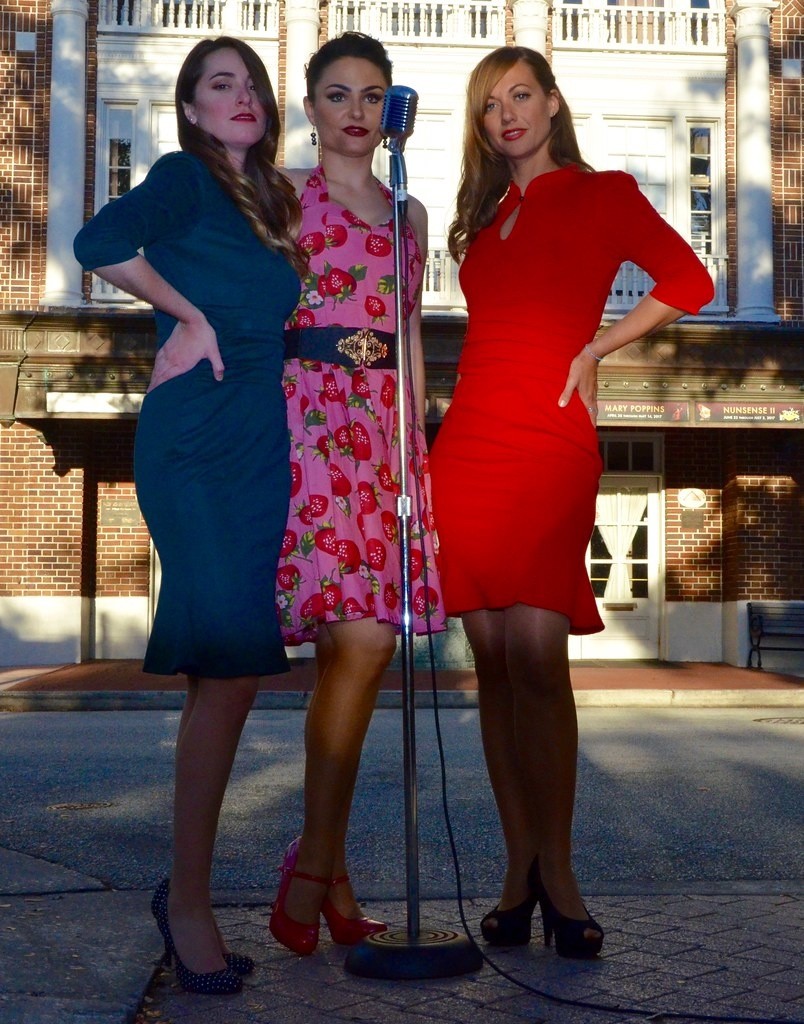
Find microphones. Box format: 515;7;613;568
378;85;419;217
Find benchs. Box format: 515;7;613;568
747;600;803;670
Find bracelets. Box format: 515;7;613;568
585;344;603;361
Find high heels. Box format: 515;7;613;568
320;871;387;947
224;951;255;977
268;835;333;954
525;852;604;960
151;879;243;994
480;869;541;948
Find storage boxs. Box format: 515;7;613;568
694;134;708;154
691;175;709;188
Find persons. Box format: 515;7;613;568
271;31;445;957
73;37;303;993
429;46;716;958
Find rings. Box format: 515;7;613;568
587;405;598;414
